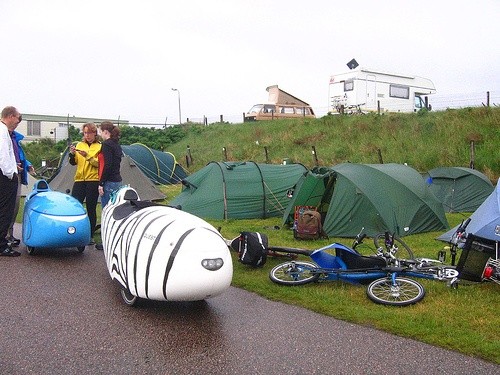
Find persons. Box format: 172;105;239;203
6;115;36;246
0;106;21;256
68;122;102;245
95;121;123;250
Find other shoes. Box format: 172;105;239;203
0;248;21;256
7;236;19;245
89;238;95;245
96;244;103;250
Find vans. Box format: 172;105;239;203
244;85;316;121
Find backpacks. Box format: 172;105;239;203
298;211;321;240
238;231;269;267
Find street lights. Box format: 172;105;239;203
171;87;182;124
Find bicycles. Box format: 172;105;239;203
216;218;500;306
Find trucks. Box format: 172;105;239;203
327;70;436;114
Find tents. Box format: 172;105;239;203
434;177;500;250
57;144;188;184
20;172;39;195
282;162;450;237
169;161;310;219
422;168;494;213
32;153;59;178
49;155;167;203
